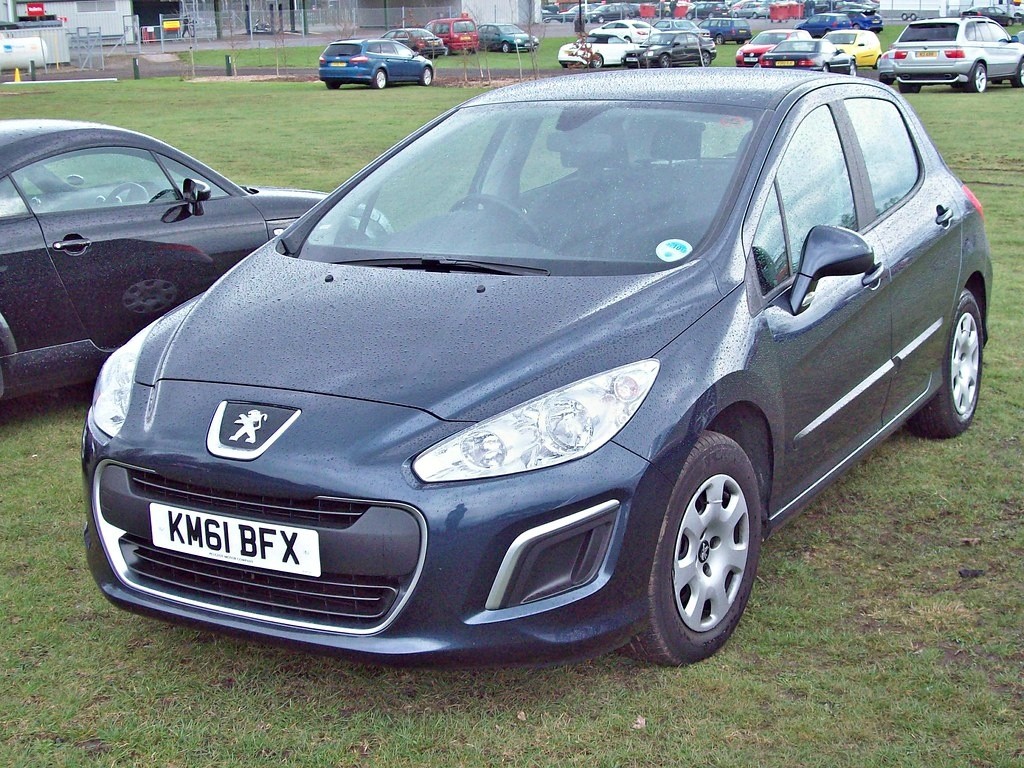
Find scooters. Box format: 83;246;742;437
254;18;271;32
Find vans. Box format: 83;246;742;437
424;18;479;56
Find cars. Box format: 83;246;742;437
630;1;769;21
653;18;710;39
735;27;813;69
794;12;852;38
319;37;435;90
475;24;540;53
559;4;596;22
835;8;884;34
584;4;632;23
590;20;661;43
878;47;897;85
558;33;641;70
621;31;717;68
82;65;993;667
820;29;882;71
0;119;397;409
801;0;880;14
962;8;1014;26
379;28;447;59
760;39;857;78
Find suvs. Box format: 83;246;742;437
696;17;752;45
890;16;1023;92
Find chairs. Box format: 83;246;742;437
511;108;752;263
